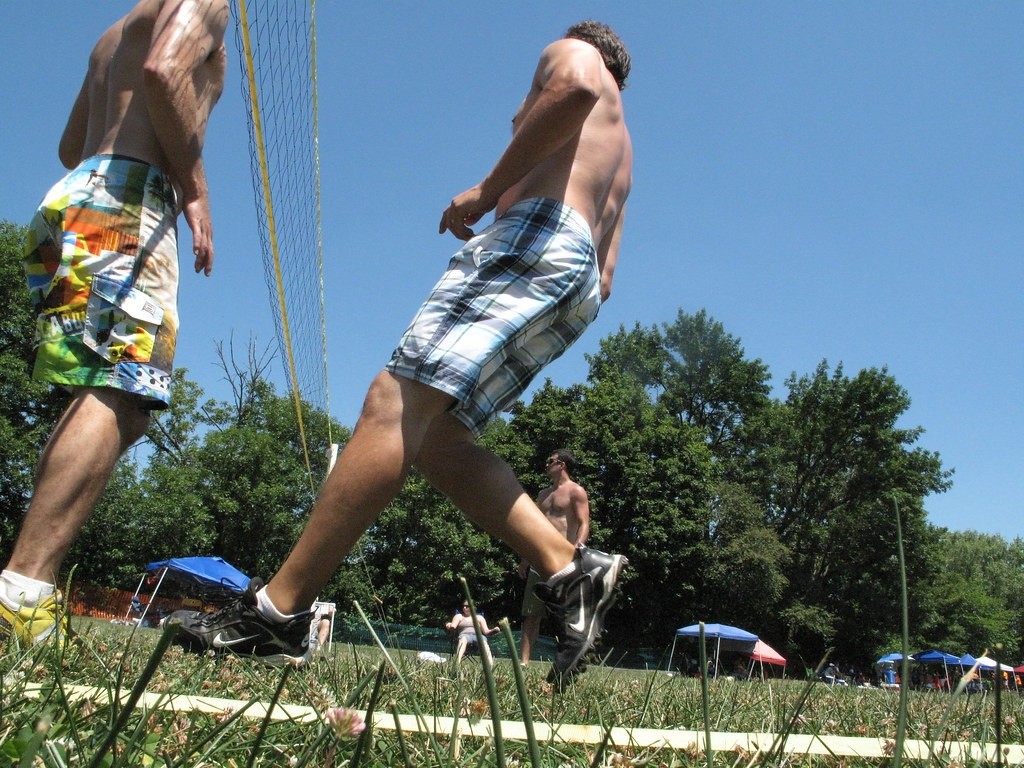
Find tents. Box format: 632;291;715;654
912;649;969;695
960;654;1024;694
669;623;758;681
748;639;787;680
126;556;252;625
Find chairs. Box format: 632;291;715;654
449;610;485;665
130;596;164;627
316;602;336;652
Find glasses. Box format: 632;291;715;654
462;604;470;608
546;459;560;464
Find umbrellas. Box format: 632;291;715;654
877;653;914;670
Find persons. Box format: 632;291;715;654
162;19;628;690
446;599;499;666
825;663;866;687
933;672;941;689
517;449;590;667
0;0;230;647
313;603;333;653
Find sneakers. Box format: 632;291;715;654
1;589;72;654
163;576;316;670
531;542;630;693
